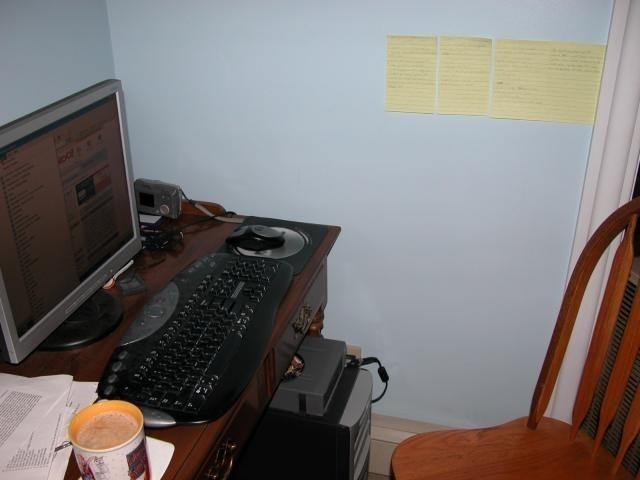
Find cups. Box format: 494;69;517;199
67;400;154;480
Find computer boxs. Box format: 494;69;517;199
227;366;373;480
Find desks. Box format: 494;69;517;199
0;212;342;478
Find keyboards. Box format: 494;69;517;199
99;253;293;427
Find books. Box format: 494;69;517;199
0;372;101;480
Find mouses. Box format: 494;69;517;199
225;225;285;251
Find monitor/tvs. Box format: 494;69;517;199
0;79;142;364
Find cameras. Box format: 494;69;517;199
134;178;182;219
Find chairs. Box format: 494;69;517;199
387;197;639;480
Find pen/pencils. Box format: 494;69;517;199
103;260;134;290
